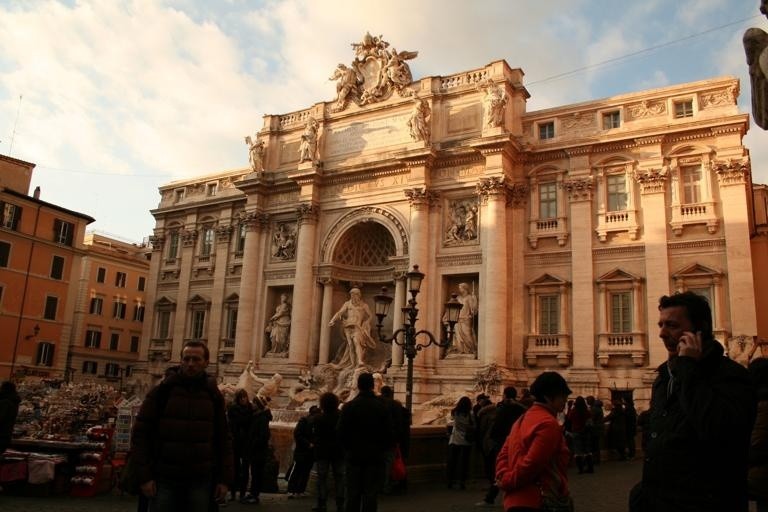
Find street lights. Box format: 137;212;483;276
373;264;463;429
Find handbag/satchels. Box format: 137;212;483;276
543;493;574;512
120;450;145;498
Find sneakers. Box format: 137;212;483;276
311;506;344;511
217;493;260;504
474;499;497;508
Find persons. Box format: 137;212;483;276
623;287;752;509
264;291;292;356
217;358;283;413
559;395;636;471
243;133;268;173
407;95;432;146
477;78;510;130
379;46;401;92
332;371;402;512
445;200;477;240
126;337;234;512
327;287;374;370
441;280;475;358
328;61;361;107
744;356;766;510
295;116;320;161
225;397;275;504
270;223;297;259
448;387;533;507
490;369;579;512
285;390;411;511
0;379;21;460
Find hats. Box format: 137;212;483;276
530;371;573;396
477;394;490;401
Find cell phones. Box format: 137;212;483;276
689;324;707;340
